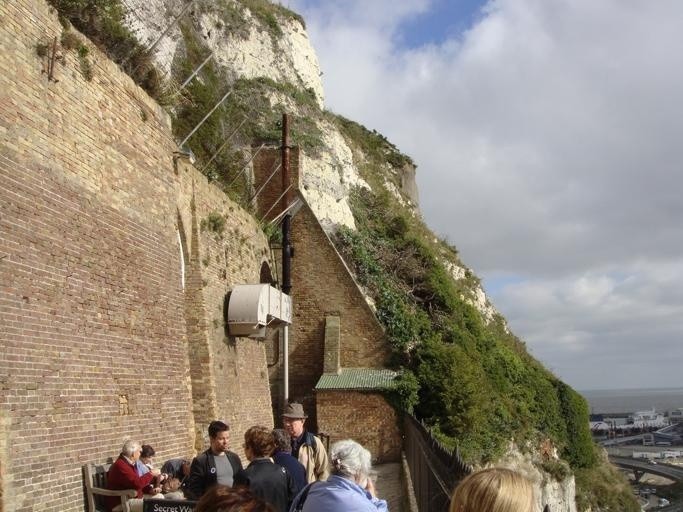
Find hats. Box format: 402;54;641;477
281;404;309;419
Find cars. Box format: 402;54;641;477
655;498;669;508
629;482;656;498
646;460;657;465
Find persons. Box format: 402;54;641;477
106;403;388;512
449;467;541;512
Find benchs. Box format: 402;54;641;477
81;461;138;511
